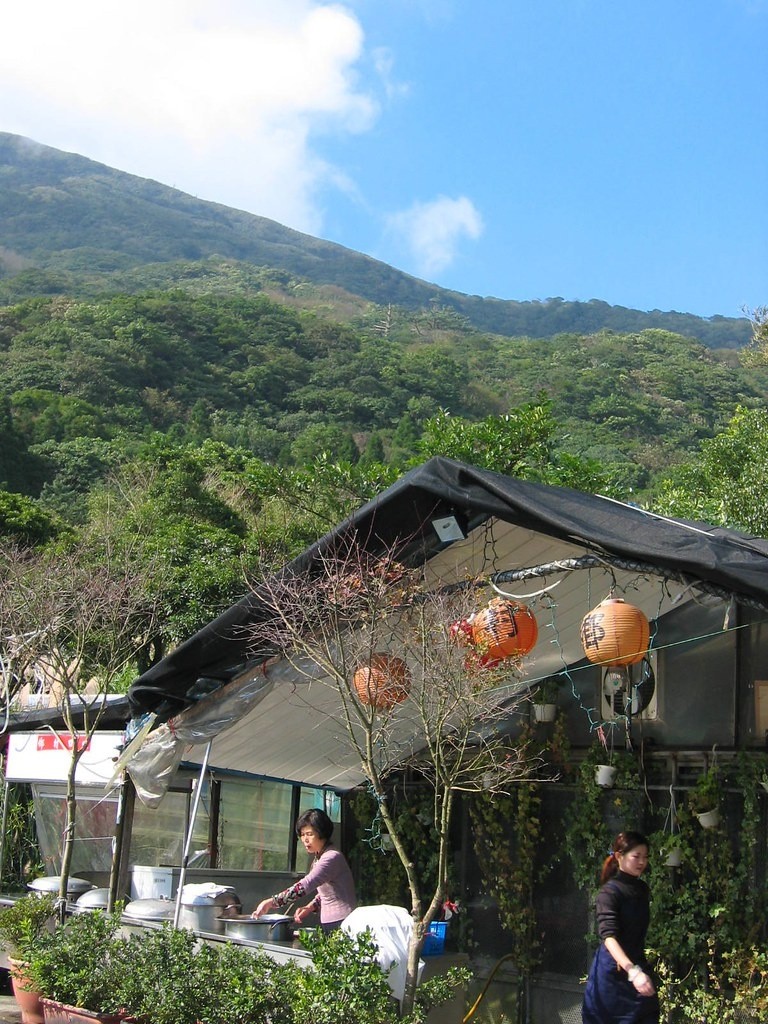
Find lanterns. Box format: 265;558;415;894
353;652;412;713
581;599;651;668
449;596;539;679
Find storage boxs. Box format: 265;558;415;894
422;921;450;956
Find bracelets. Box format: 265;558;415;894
627;965;643;982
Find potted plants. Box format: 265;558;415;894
0;678;768;1024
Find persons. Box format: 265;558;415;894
250;808;357;945
579;831;662;1024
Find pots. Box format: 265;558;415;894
26;874;97;903
214;913;295;942
122;894;175;921
76;888;117;909
175;882;244;934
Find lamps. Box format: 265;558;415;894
431;506;470;543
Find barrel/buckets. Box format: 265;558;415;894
131;865;172;900
421;921;449;956
